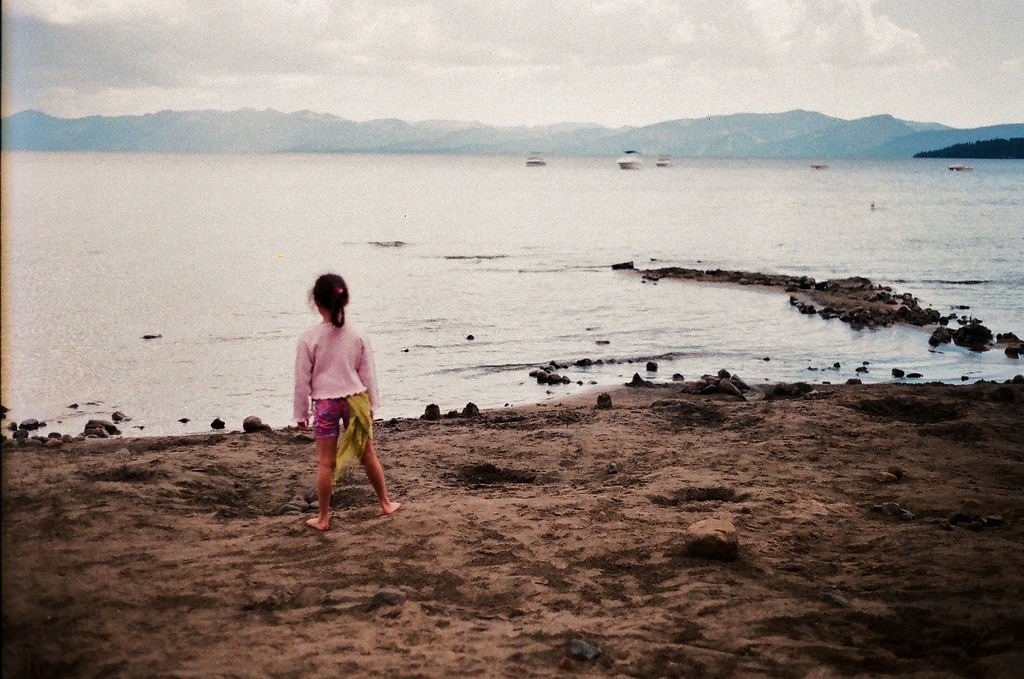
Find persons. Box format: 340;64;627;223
295;274;401;530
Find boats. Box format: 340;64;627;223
526;150;546;165
655;155;671;168
812;161;829;171
617;150;646;171
